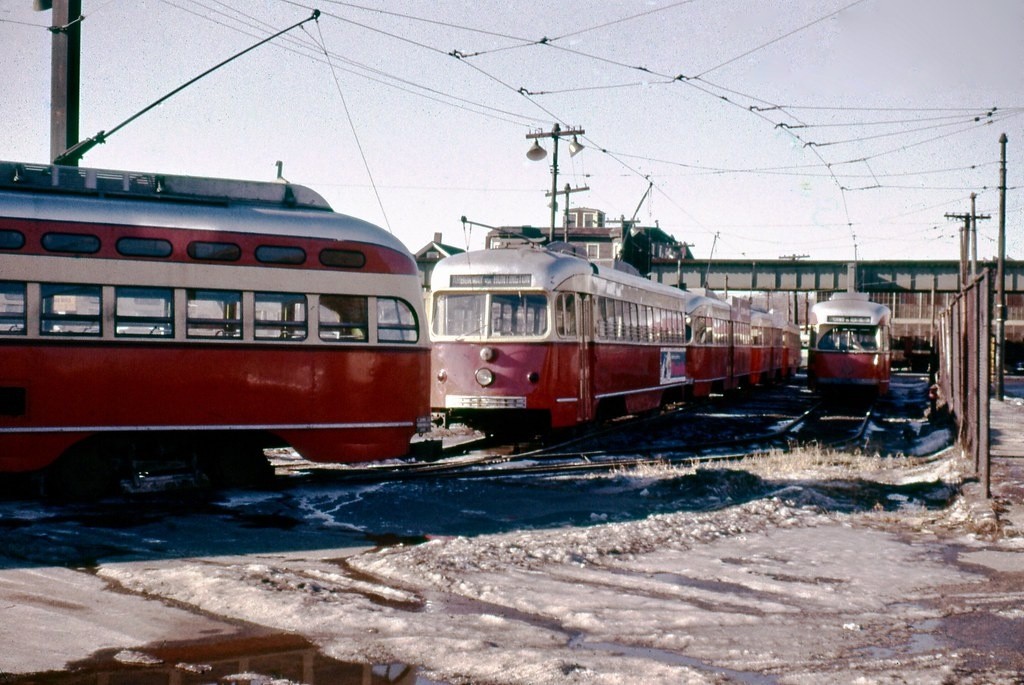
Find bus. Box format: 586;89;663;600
808;293;891;398
782;322;803;376
1;161;431;470
686;291;753;397
751;306;783;384
430;241;693;432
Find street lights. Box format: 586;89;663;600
526;129;585;242
